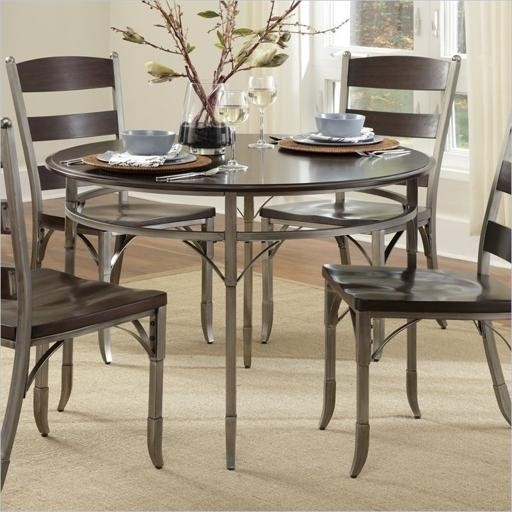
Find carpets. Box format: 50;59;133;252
0;268;511;512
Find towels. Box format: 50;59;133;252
108;144;183;167
310;127;374;142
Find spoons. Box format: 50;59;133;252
167;168;220;180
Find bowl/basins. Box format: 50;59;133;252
316;113;366;137
119;129;176;155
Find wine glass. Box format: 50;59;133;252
247;69;278;149
215;90;249;172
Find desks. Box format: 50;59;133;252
44;134;433;472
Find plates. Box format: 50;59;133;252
95;151;197;165
290;132;384;146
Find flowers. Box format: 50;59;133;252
109;0;352;124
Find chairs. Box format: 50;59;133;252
259;49;462;360
1;116;168;492
319;107;512;478
4;50;216;365
